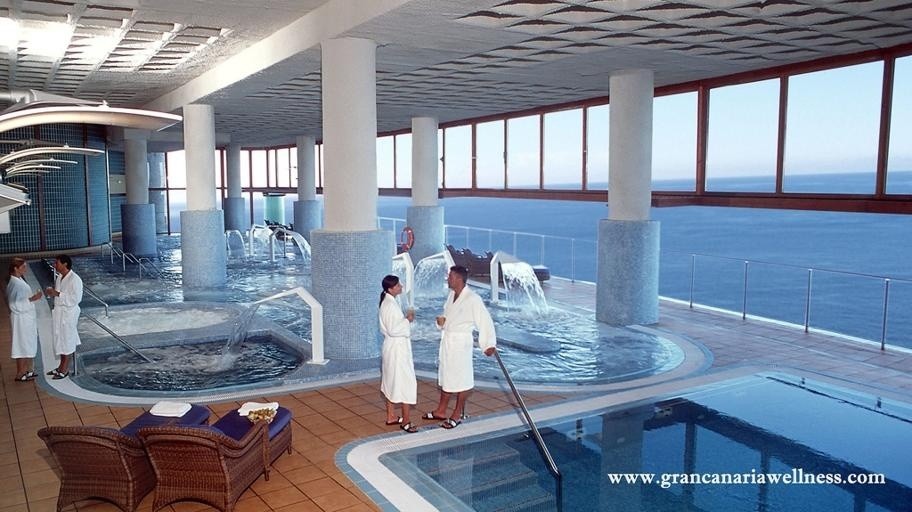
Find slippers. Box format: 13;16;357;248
442;418;461;430
385;416;419;433
14;371;38;381
422;411;447;421
46;368;69;380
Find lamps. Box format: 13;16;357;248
0;90;183;132
0;153;79;179
0;138;105;165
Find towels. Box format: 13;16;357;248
238;401;280;420
149;400;192;418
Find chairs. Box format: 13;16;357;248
447;242;550;288
37;403;210;511
265;219;295;243
135;406;292;511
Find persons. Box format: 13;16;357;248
45;254;84;379
378;275;418;433
422;265;497;429
5;258;43;381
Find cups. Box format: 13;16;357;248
46;286;53;299
36;288;43;300
437;314;446;329
406;306;416;322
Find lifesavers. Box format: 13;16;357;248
400;228;413;252
